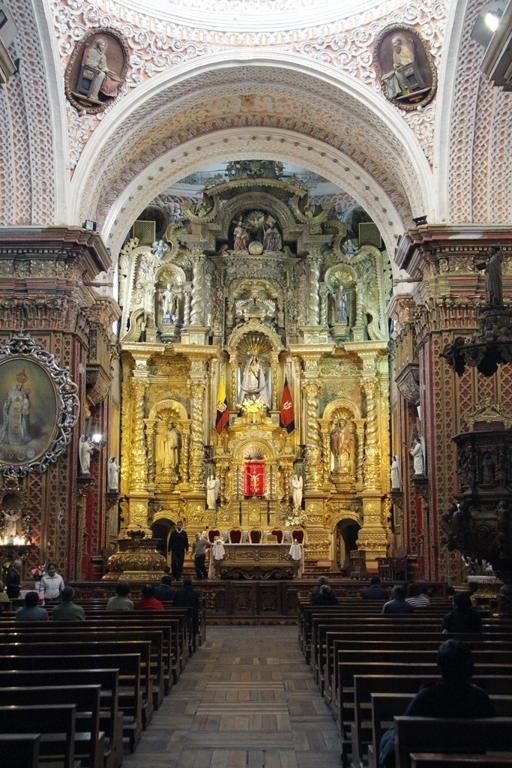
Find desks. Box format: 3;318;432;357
207;543;305;580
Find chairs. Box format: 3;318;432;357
291;529;304;545
208;529;220;542
228;529;242;543
270;529;283;543
248;529;262;544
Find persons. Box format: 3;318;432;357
161;421;179;472
334;284;347;323
391;455;400;492
160;282;176;324
3;380;31;446
292;475;304;509
409;437;423;476
311;575;486;634
484;245;503;305
2;509;20;537
110;457;121;493
262;215;282;252
379;638;493;768
331;414;354;475
207;475;218;510
0;522;218;634
234;220;249;250
83;40;108;101
82;435;94;474
392;36;415;95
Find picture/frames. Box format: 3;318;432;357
0;328;81;480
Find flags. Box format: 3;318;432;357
215;363;229;435
279;377;294;434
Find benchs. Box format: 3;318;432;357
298;596;512;768
0;598;206;768
